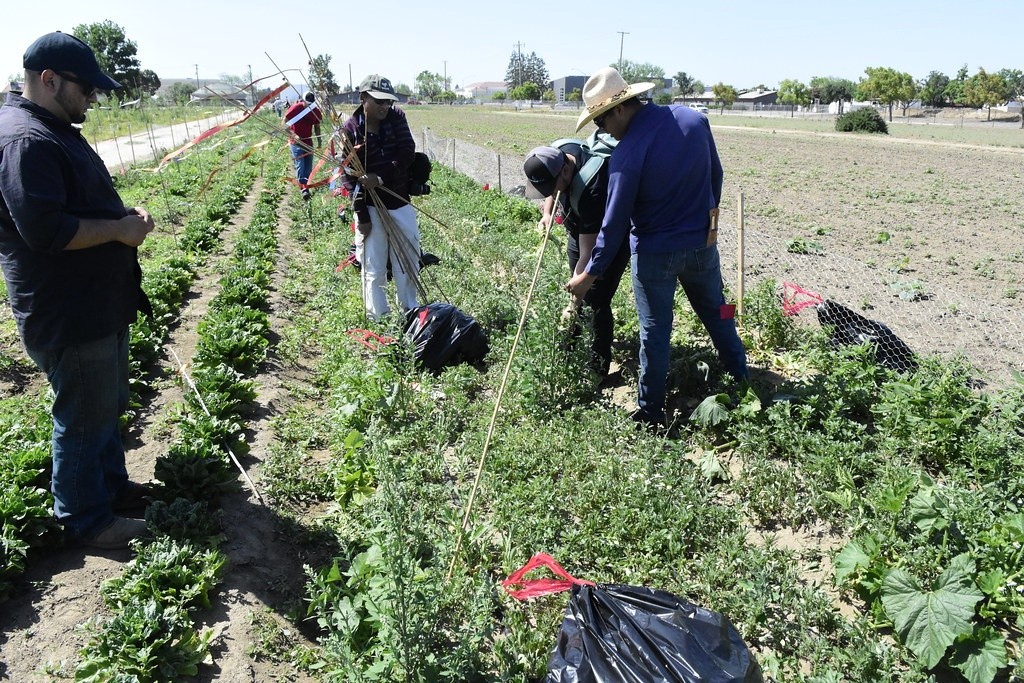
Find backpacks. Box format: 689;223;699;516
567;128;621;216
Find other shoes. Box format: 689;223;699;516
85;514;150;551
301;188;311;199
629;411;667;438
115;496;157;518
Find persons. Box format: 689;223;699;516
337;74;421;329
282;92;323;198
270;95;303;119
521;138;633;386
563;68;751;436
0;29;167;546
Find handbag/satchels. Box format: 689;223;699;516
394;303;489;374
409;152;432;185
504;557;765;683
782;281;917;376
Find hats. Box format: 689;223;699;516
575;67;656;133
523;145;569;199
304;91;315;104
359;74;399;101
23;30;122;90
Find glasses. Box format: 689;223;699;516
38;66;96;97
365;95;393;105
594;110;613;127
